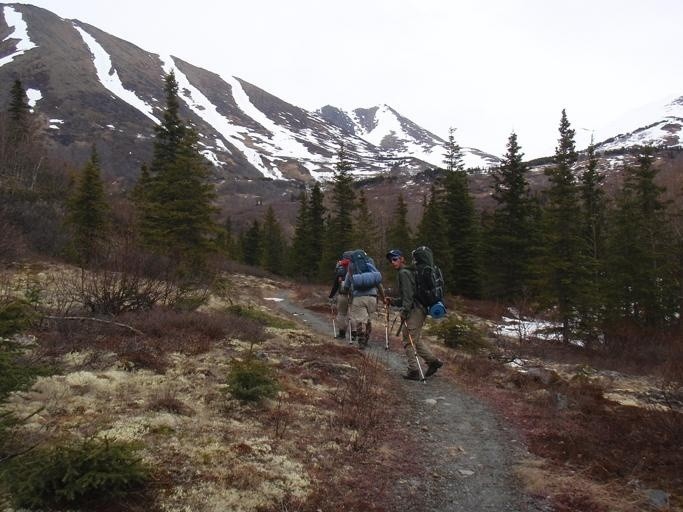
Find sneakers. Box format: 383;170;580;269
333;330;371;351
402;372;419;381
426;359;443;377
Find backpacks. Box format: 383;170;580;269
402;246;444;307
348;251;382;292
335;259;352;294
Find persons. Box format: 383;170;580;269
344;250;386;351
383;250;443;381
329;251;357;341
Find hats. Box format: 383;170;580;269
387;249;401;259
343;251;353;258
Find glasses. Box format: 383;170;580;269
390;257;400;263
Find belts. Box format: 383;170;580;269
362;295;376;297
